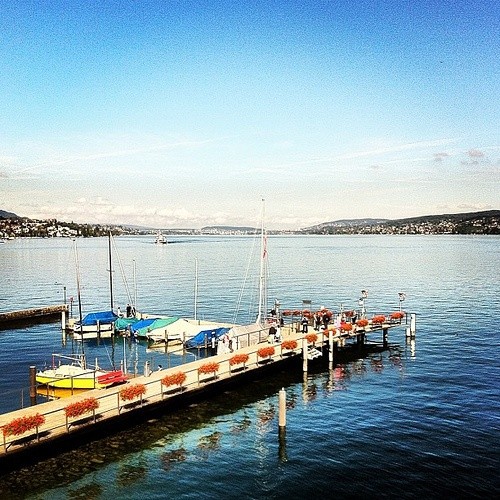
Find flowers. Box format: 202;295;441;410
230;354;249;365
197;361;220;375
160;370;187;386
64;397;100;417
257;347;275;358
120;383;147;401
281;309;404;350
1;412;46;437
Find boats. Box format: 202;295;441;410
153;230;168;244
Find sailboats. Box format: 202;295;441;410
68;199;281;355
34;239;128;389
35;384;91;399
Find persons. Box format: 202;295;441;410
268;324;281;344
223;331;234;353
158;365;163;370
340;316;346;333
314;312;330;331
118;304;131;317
301;317;309;334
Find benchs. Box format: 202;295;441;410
97;370;128;389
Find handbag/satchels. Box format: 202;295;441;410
268;335;272;342
